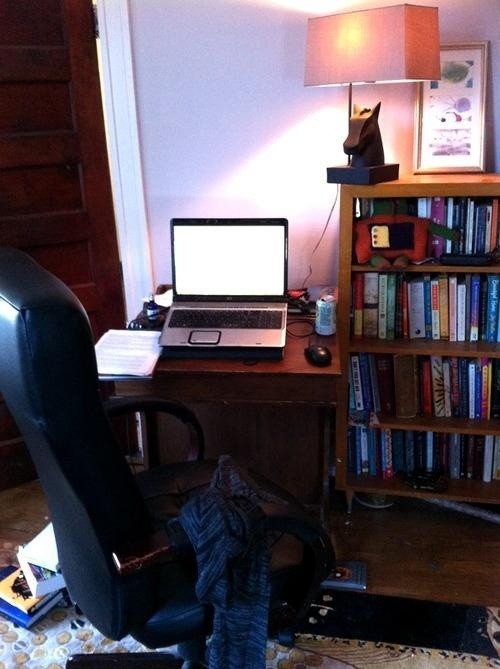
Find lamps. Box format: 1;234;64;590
303;4;442;185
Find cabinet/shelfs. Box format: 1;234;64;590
336;174;500;504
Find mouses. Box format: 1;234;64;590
304;344;332;366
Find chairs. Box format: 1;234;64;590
0;252;339;669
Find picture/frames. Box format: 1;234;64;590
413;40;490;173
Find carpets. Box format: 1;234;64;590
0;579;499;669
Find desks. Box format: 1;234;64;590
104;314;340;535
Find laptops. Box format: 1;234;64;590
158;217;288;348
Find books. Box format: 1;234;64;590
0;563;64;628
94;327;163;380
351;196;500;266
353;426;499;482
349;353;500;421
349;271;498;343
